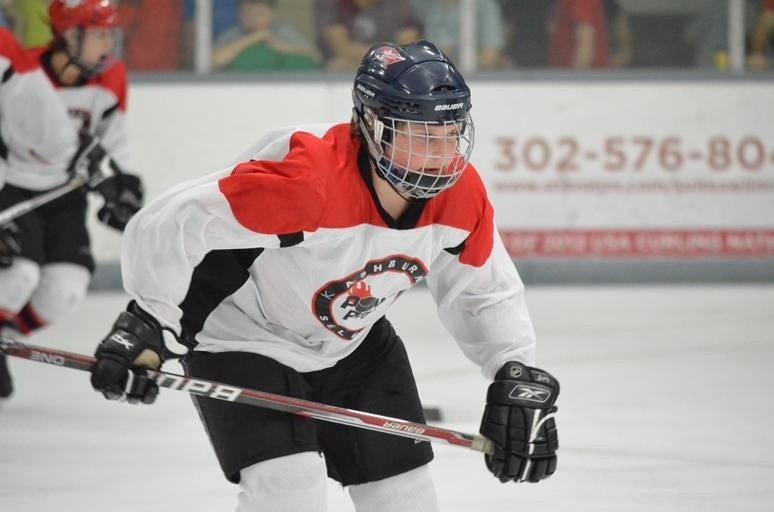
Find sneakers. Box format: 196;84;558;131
0;354;12;398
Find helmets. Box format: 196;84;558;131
49;1;125;79
351;40;475;200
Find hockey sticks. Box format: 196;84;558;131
0;338;494;466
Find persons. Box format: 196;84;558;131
0;0;773;76
0;0;143;398
91;39;561;512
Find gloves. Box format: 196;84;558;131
91;298;188;405
99;176;143;232
480;361;559;484
1;220;23;267
